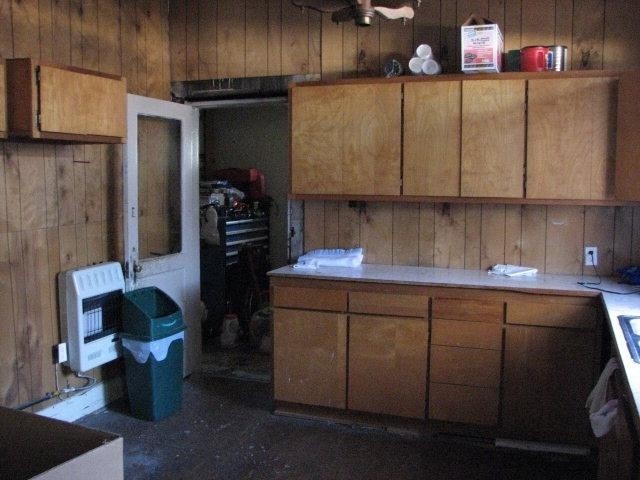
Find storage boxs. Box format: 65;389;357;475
0;405;126;479
460;13;505;73
200;193;225;207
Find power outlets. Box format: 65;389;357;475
585;246;598;266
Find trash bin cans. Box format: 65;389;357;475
119;286;187;422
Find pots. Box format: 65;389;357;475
519;44;569;71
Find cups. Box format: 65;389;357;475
409;44;442;74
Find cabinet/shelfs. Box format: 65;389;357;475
266;277;431;419
285;68;639;207
503;289;607;449
429;287;506;428
597;379;640;480
200;195;274;345
0;57;127;146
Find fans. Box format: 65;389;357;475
294;0;415;28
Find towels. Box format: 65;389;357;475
584;357;618;414
488;262;538;278
588;398;618;438
293;259;320;270
